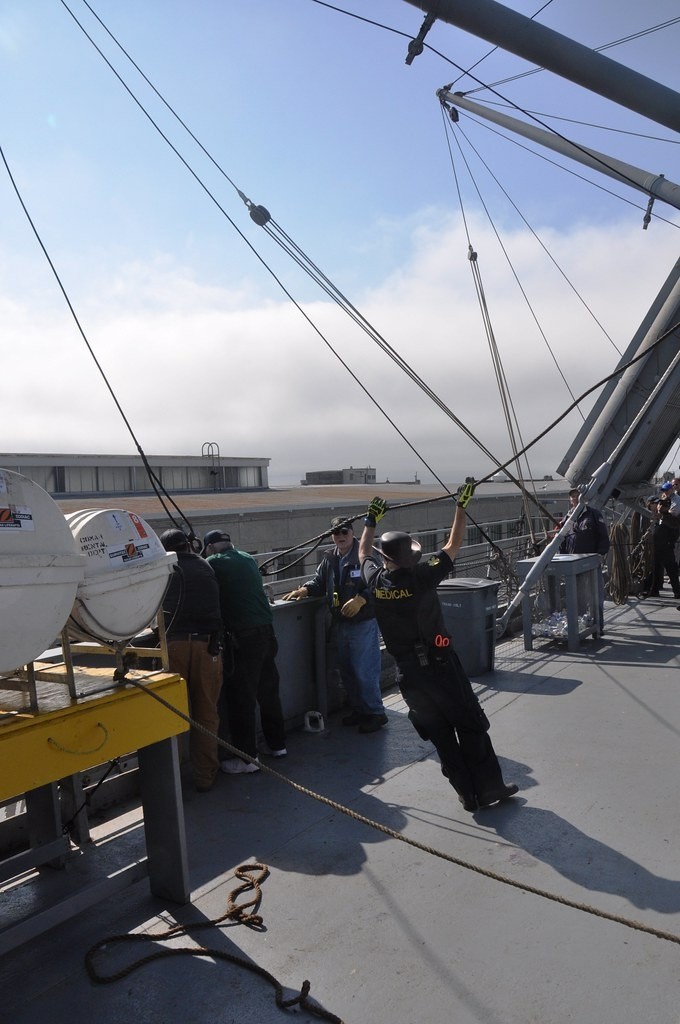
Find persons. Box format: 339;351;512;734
283;516;389;735
643;478;680;600
146;528;225;793
196;528;288;774
550;488;610;635
358;477;520;811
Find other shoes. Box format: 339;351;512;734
196;775;220;791
642;571;680;612
478;781;518;805
457;793;478;811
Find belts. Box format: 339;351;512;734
166;634;213;641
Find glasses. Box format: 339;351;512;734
333;527;350;535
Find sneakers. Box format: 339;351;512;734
258;742;288;758
344;710;362;725
221;756;260;774
360;712;389;732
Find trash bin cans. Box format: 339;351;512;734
436;577;502;676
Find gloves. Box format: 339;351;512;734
364;496;389;528
456;476;476;509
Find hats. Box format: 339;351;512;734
568;488;579;495
162;528;190;550
659;497;671;507
660;483;673;491
647;495;660;503
198;529;232;556
372;530;422;568
